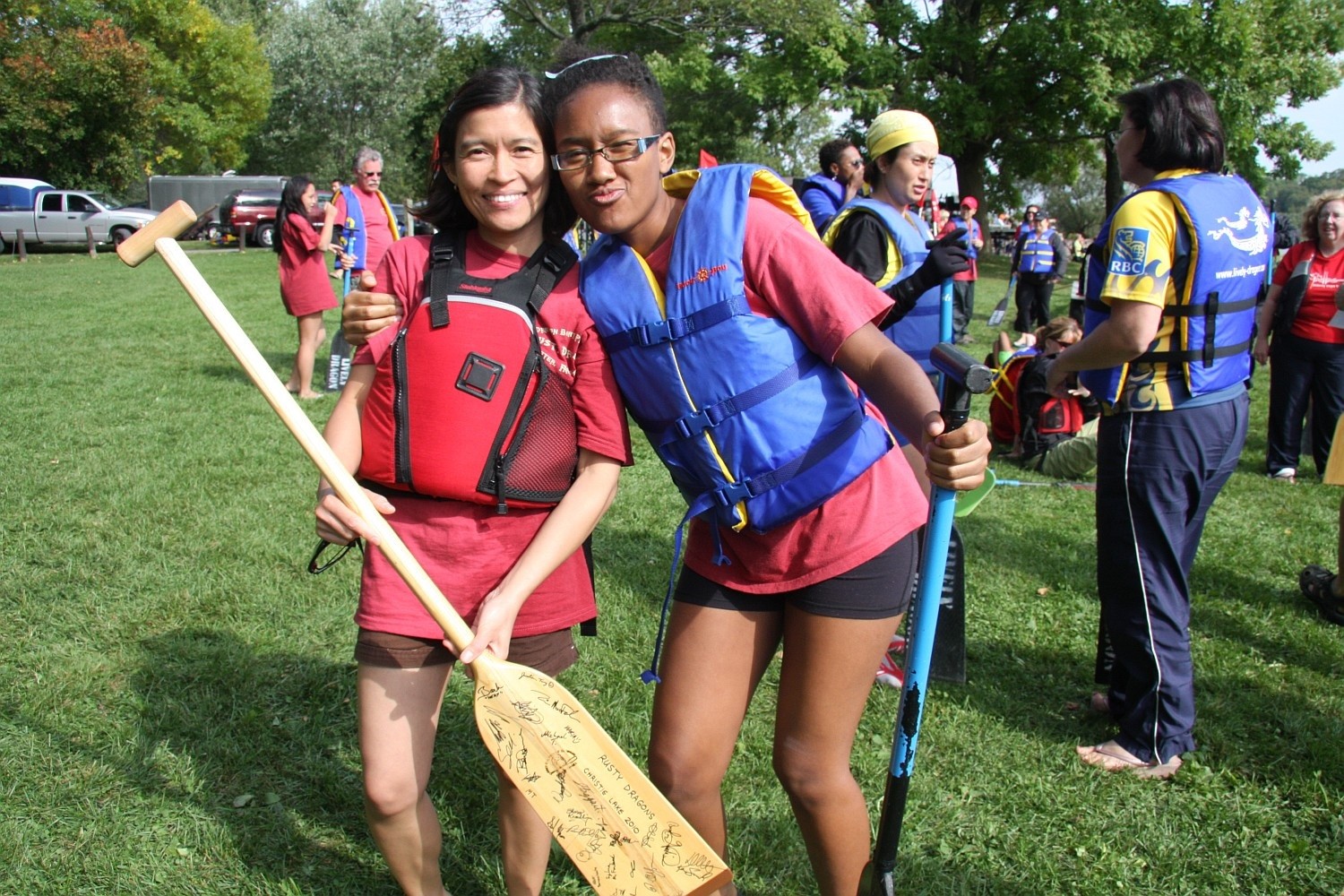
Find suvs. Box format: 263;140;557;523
218;186;336;248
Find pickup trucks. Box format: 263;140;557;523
0;190;160;255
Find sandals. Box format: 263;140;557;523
1300;564;1344;626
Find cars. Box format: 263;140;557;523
389;203;438;239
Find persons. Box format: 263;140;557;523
986;204;1098;479
271;148;406;400
1299;495;1344;627
315;61;635;896
1044;80;1273;779
340;52;992;896
792;110;984;690
1244;190;1343;479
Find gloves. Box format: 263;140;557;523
898;227;971;304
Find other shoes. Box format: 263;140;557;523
1274;466;1295;477
873;652;904;691
887;634;907;654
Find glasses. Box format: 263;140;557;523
838;158;864;170
962;206;971;211
1106;125;1147;144
546;134;663;171
360;170;384;178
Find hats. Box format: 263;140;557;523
961;197;977;209
867;110;940;161
1034;212;1050;221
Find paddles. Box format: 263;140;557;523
1323;413;1344;487
902;238;972;684
116;200;737;894
985;275;1017;327
325;225;361;393
991;469;1096;493
856;340;995;896
1266;197;1277;293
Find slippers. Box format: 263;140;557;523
1088;689;1112;711
1079;740;1181;779
